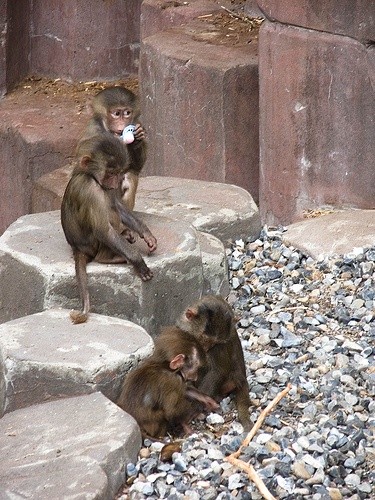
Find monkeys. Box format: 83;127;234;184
114;293;254;441
57;86;159;324
